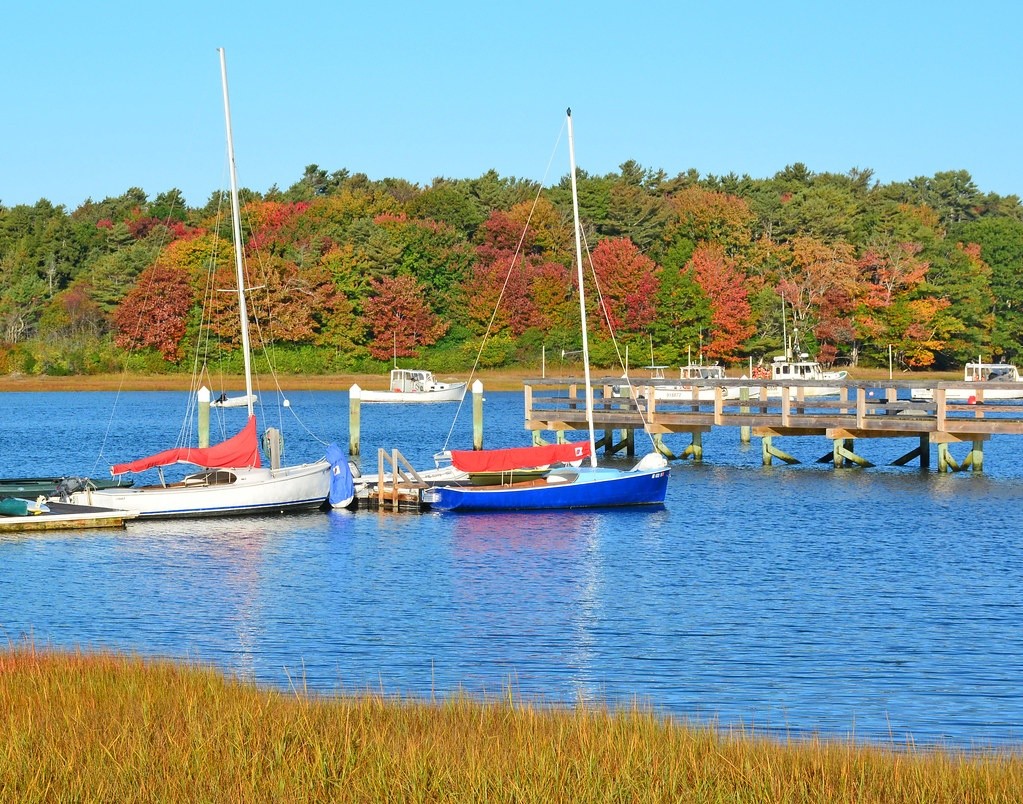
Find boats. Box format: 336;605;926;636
210;395;257;407
749;291;848;397
612;326;761;400
1;476;136;501
911;354;1023;401
360;331;468;404
354;458;584;483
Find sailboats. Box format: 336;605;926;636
421;107;671;513
50;49;333;522
